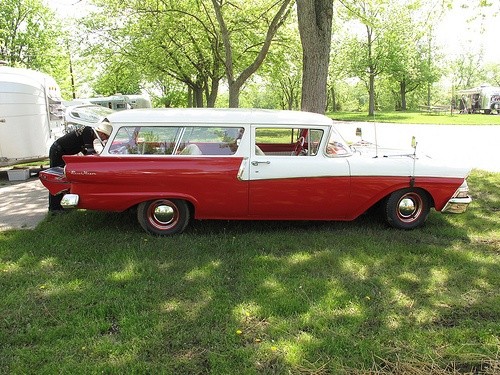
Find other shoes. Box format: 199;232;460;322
48;208;68;216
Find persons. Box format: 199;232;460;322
48;122;113;215
459;94;477;114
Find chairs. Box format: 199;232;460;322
237;139;265;156
180;144;202;155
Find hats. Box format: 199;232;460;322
96;122;113;136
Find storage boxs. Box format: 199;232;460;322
6;167;29;180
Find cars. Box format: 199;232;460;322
36;105;472;238
